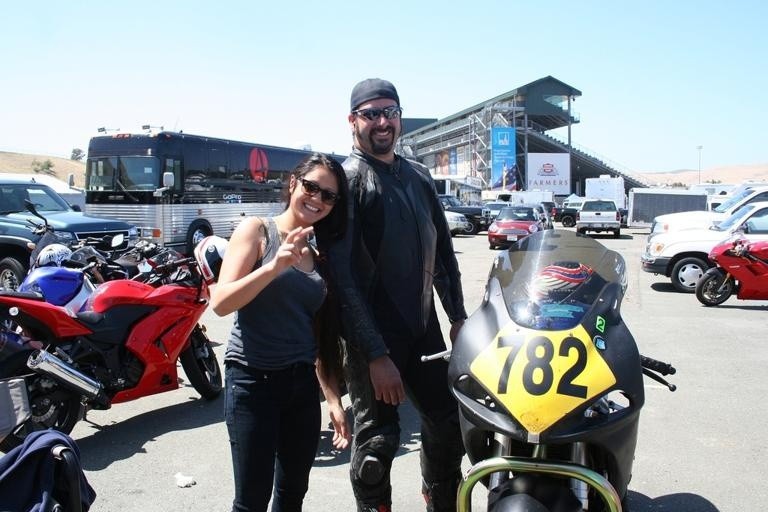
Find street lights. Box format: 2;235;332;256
696;146;703;184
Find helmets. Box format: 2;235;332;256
36;242;72;267
527;258;610;311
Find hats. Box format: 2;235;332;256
347;77;400;110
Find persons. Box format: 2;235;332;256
322;78;468;512
211;151;351;512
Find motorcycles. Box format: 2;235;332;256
415;226;679;510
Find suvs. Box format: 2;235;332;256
0;179;136;295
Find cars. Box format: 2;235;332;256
433;177;628;251
641;184;767;305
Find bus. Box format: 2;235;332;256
83;126;349;259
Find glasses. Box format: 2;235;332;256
352;105;401;119
296;177;342;207
